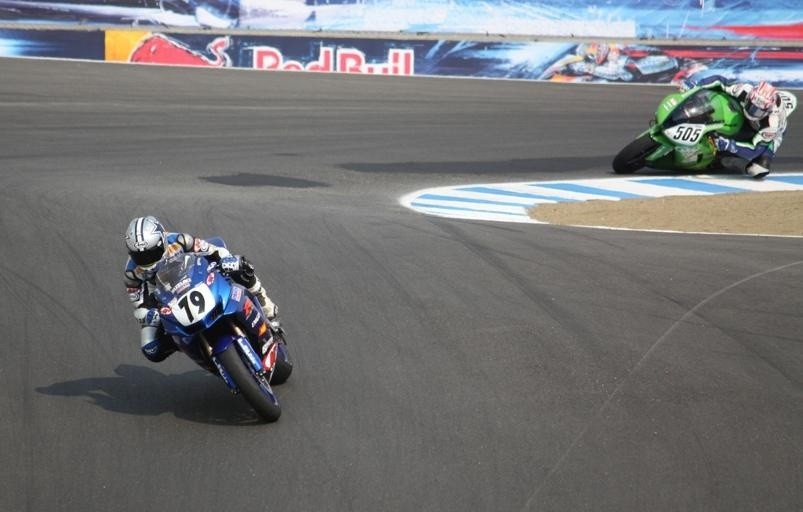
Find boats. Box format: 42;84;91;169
534;42;679;84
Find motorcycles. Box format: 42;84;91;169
133;234;294;423
611;76;797;174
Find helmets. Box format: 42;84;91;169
741;80;779;122
124;216;168;273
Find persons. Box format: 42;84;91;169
683;68;786;180
122;215;278;363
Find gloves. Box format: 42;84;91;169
218;257;239;273
678;79;699;93
713;136;738;154
147;309;162;326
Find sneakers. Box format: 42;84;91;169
746;162;770;179
255;286;278;320
